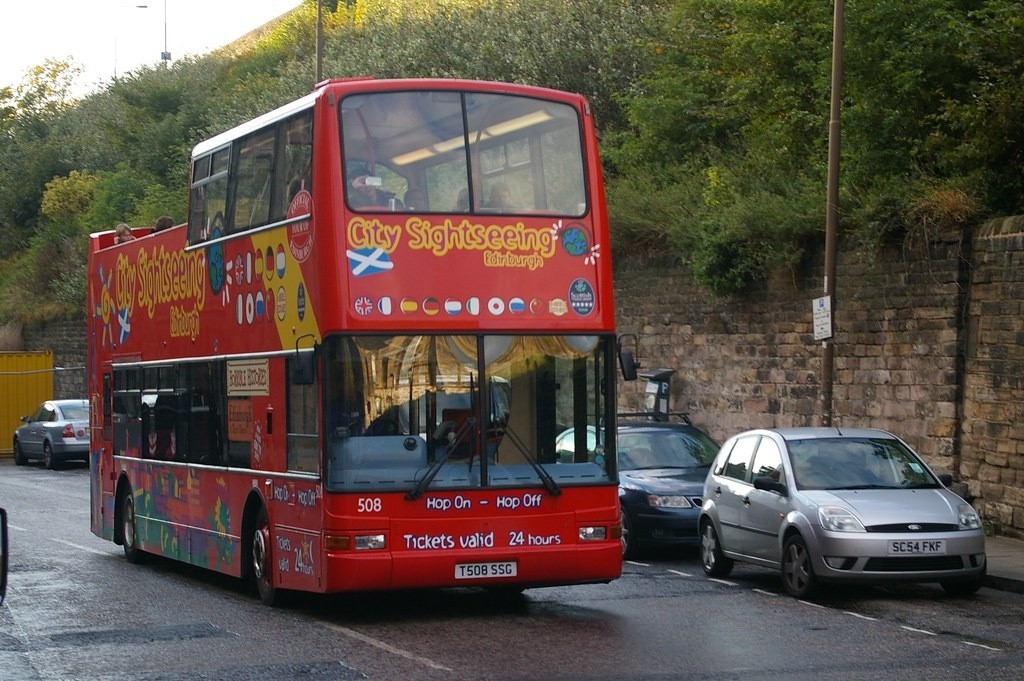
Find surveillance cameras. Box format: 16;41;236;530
404;438;417;450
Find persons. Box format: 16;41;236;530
113;224;136;245
484;180;518;210
455;186;473;212
150;216;175;237
346;168;404;209
403;187;428;211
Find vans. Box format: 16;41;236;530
375;372;515;463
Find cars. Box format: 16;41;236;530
695;424;990;607
552;418;722;568
13;400;91;470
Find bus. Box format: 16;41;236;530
88;70;626;609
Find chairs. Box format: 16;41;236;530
121;392;426;475
